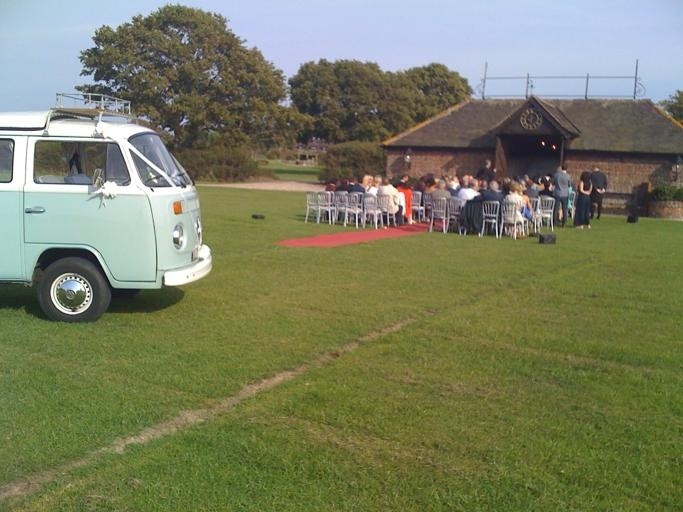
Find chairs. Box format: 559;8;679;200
302;191;576;241
37;173;92;185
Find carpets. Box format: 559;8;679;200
273;215;446;250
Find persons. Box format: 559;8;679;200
573;171;594;230
322;158;579;240
589;165;607;219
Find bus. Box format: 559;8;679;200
0;90;215;323
